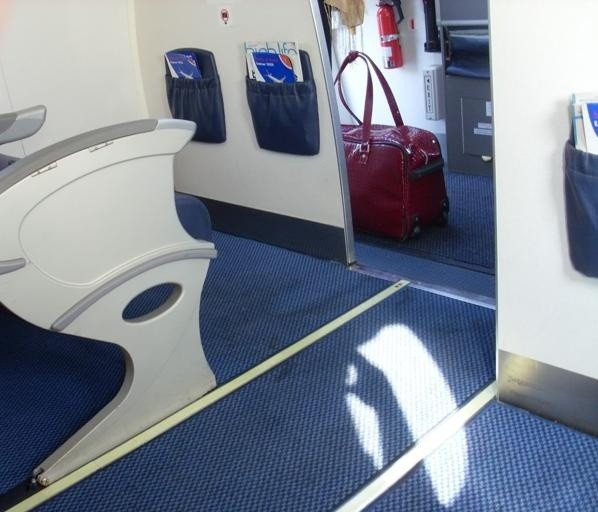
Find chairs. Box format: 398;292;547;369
1;106;219;498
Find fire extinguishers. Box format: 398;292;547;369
376;0;403;69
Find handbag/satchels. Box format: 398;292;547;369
334;51;451;246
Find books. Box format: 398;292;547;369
165;51;202;79
245;41;304;83
571;91;598;154
